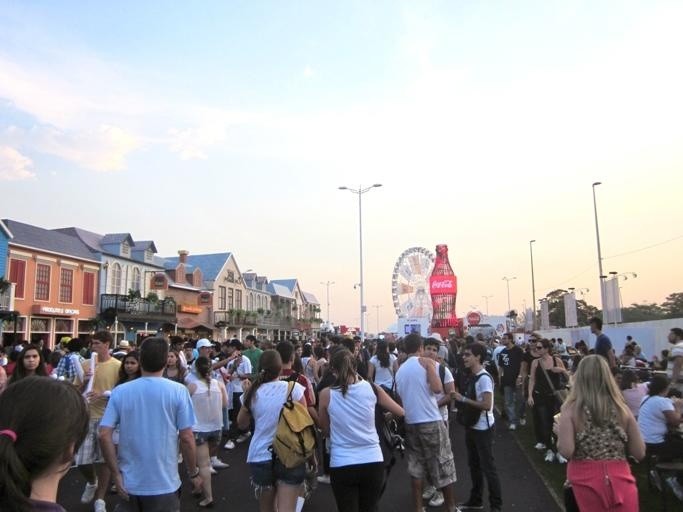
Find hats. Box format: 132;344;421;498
427;332;445;343
196;338;216;350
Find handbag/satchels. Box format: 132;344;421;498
370;381;407;495
553;389;569;407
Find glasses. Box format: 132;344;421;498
462;352;470;358
527;339;536;343
536;347;541;349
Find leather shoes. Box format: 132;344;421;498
195;500;214;507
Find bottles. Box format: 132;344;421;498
427;243;458;326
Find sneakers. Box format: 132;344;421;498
649;470;662;492
209;429;253;474
509;424;515;431
664;476;682;502
534;443;567;464
317;474;331;485
94;499;107;512
519;417;526;426
81;478;99;504
422;485;483;512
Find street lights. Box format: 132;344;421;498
143;321;150;336
265;327;269;339
480;295;494;314
223;326;230;338
592;180;610;328
468;304;478;311
319;280;335;322
277;327;281;341
529;236;538;331
502;276;519;313
340;182;382;334
113;318;121;349
174;321;179;336
239;328;243;342
252;328;255;336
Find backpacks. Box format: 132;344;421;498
268;381;318;469
455;373;490;427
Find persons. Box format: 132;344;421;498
1;318;683;511
